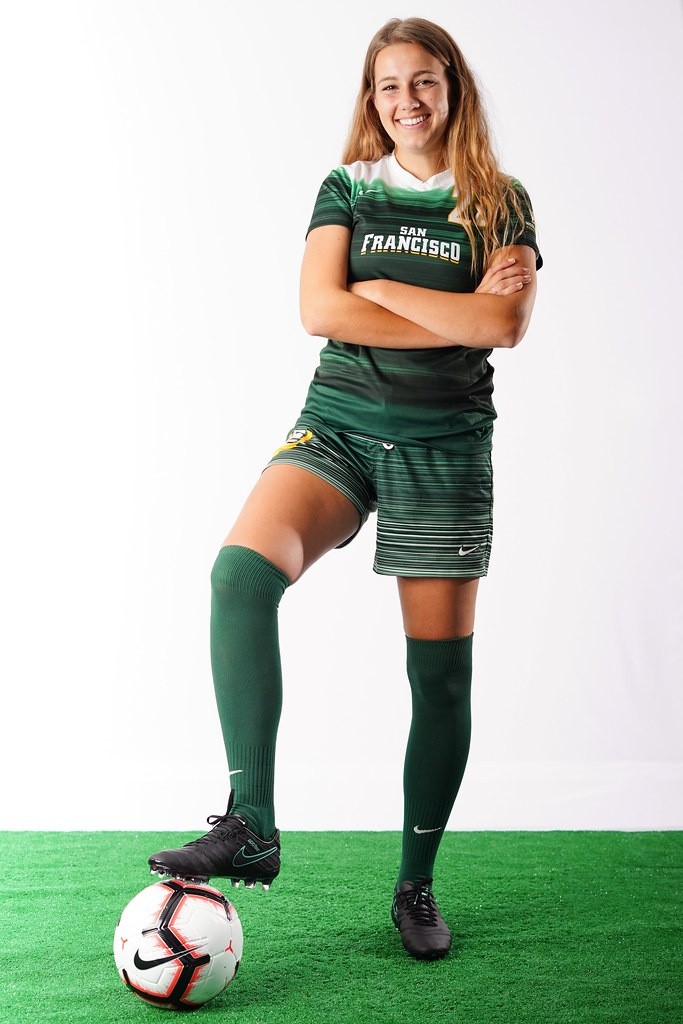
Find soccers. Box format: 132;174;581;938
112;878;244;1011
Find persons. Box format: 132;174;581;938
149;18;544;962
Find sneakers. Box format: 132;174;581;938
391;878;452;958
143;788;281;891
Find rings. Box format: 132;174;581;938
491;288;498;294
